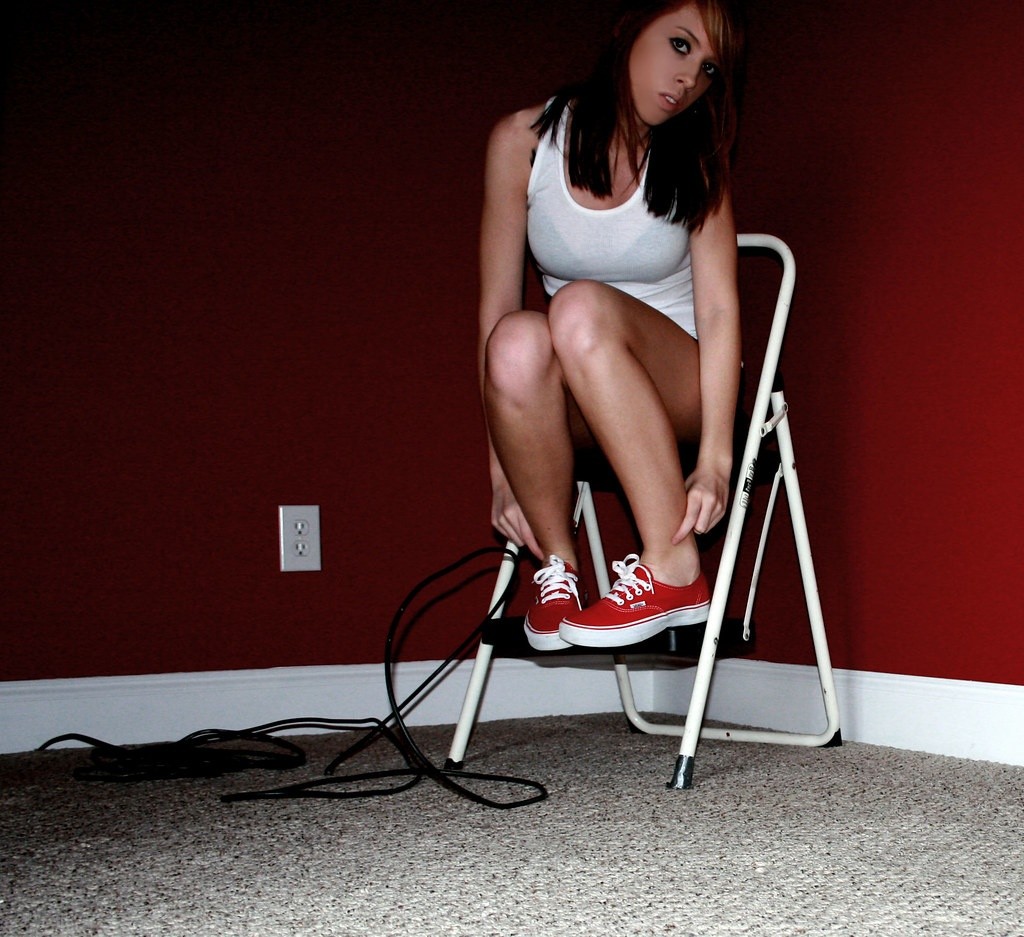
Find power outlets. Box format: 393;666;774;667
280;505;321;572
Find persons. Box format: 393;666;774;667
477;0;756;651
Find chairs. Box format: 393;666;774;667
445;234;841;789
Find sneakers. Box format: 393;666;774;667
524;555;586;651
559;553;712;648
